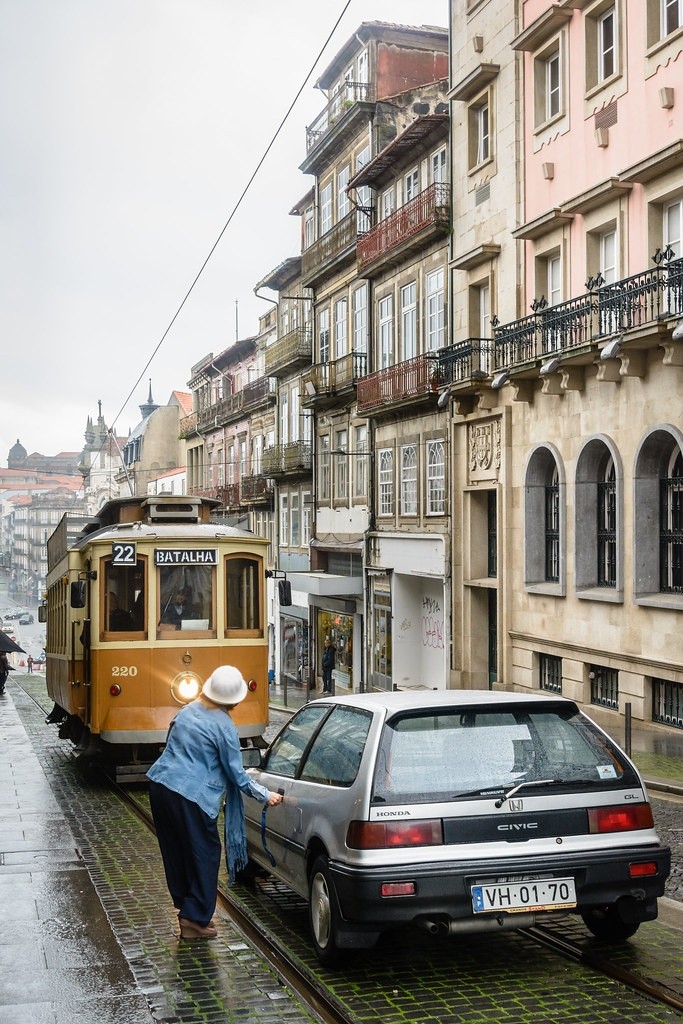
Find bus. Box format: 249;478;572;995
38;491;293;783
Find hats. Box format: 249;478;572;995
201;665;248;705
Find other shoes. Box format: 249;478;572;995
319;690;324;693
323;691;331;694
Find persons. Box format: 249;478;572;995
108;591;136;631
164;590;192;626
27;655;33;673
145;666;282;938
321;639;336;694
0;653;9;695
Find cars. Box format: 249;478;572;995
222;690;671;973
32;652;46;665
19;614;34;625
4;609;29;620
1;621;15;634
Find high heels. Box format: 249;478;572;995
177;916;218;938
